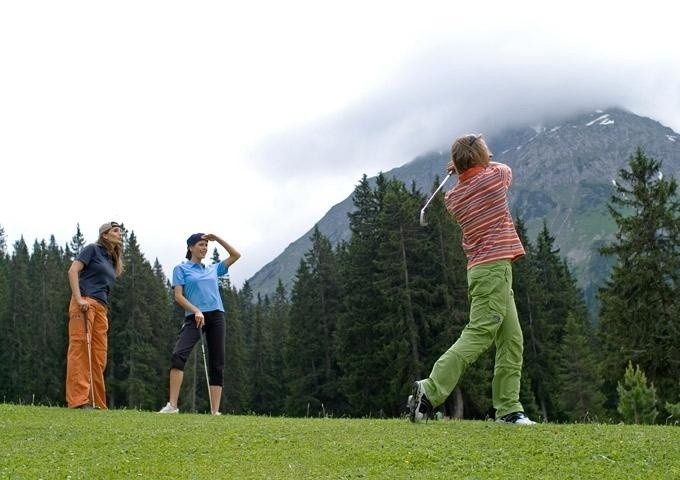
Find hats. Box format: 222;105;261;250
187;233;208;258
99;221;121;235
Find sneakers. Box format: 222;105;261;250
80;403;101;409
409;380;434;424
159;401;180;414
494;411;536;425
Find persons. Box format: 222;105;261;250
159;232;240;414
410;135;540;425
65;222;123;410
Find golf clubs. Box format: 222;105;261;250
84;311;100;409
200;328;221;416
419;170;454;226
